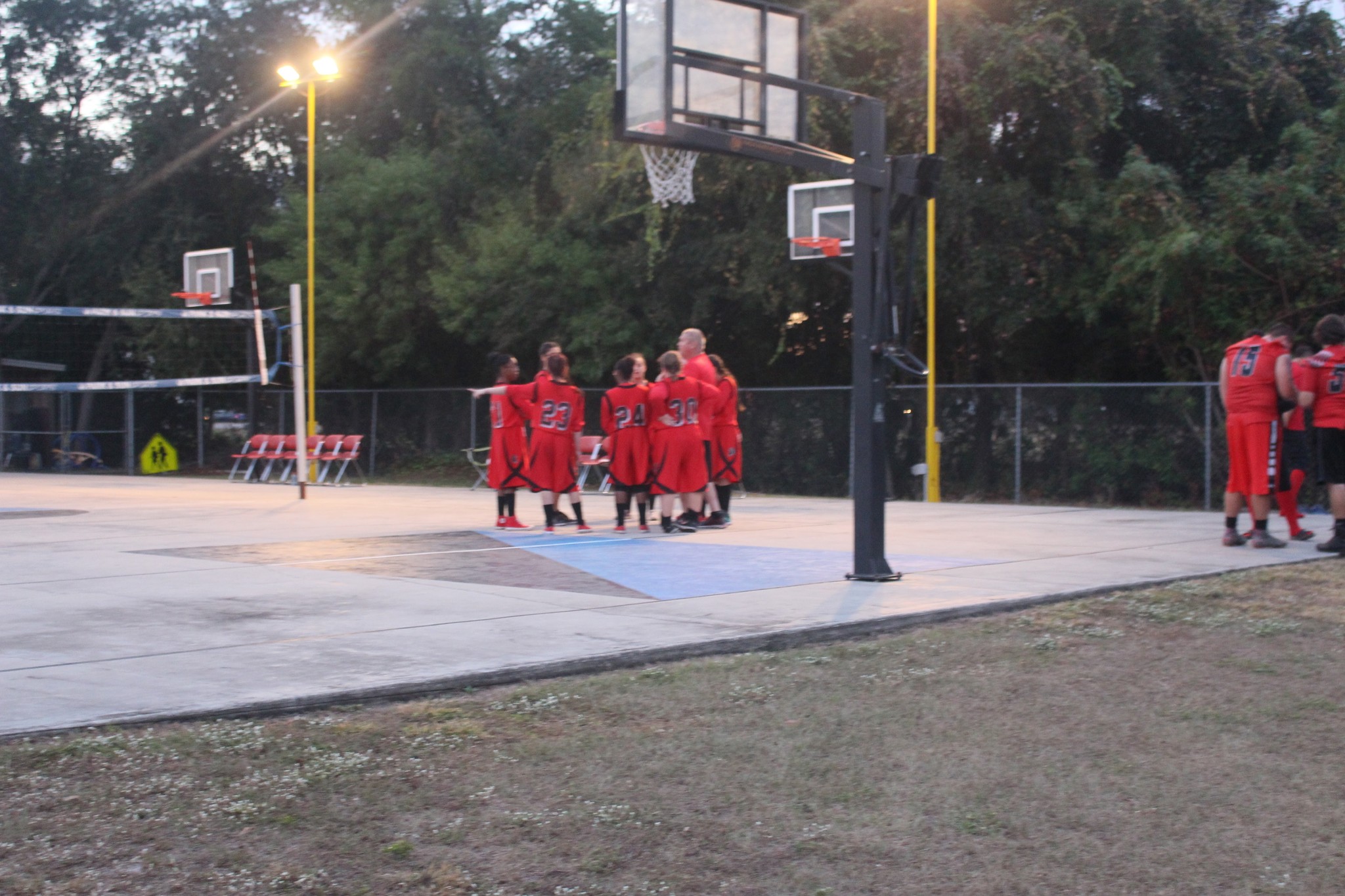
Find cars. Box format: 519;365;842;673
213;408;265;449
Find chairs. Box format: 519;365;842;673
463;436;610;492
230;434;364;485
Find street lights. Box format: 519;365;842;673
275;54;343;482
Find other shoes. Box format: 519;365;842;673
697;510;727;528
664;524;679;533
1252;528;1285;547
649;513;657;520
613;525;626;533
552;512;577;525
616;515;630;520
1242;529;1253;537
578;525;591;532
1291;529;1313;540
1222;528;1248;544
506;516;531;531
726;513;731;524
672;517;698;531
544;526;555;533
1317;520;1345;551
640;524;649;531
497;515;507;529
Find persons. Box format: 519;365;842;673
599;328;742;535
534;341;578;527
489;354;538;531
1217;313;1345;560
465;352;593;533
